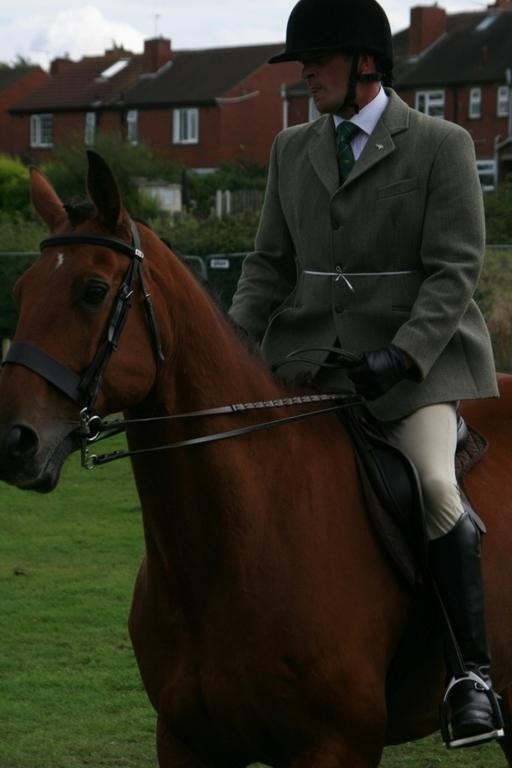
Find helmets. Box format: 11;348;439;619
268;0;393;72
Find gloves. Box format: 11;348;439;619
344;347;405;401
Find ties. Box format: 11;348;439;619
335;121;361;179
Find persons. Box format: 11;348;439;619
227;0;505;749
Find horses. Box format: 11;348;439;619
0;149;511;767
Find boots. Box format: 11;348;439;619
421;511;497;739
